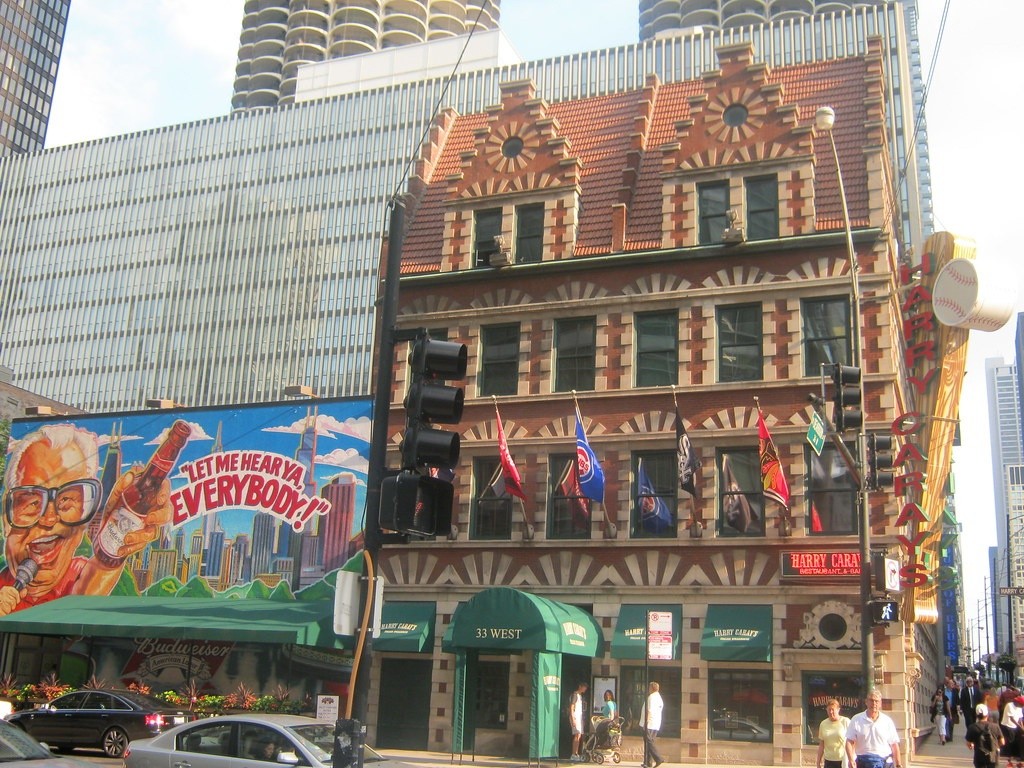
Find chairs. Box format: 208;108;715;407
270;732;286;749
242;730;262;760
68;699;80;709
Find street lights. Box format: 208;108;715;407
971;612;1009;666
966;625;984;667
977;595;1024;679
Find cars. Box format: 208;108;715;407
710;718;770;741
123;714;419;768
0;718;102;768
3;690;196;758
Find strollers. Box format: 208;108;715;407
580;716;625;764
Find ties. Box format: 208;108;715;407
970;688;973;708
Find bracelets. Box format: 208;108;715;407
896;764;903;767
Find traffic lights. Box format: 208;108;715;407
373;330;468;540
869;600;899;625
831;362;862;432
867;433;894;489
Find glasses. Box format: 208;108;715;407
937;691;942;693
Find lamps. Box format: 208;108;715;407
815;106;835;130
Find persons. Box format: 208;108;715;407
253;737;278;762
602;689;617;720
569;676;590;762
639;682;665;768
929;676;1024;768
844;690;904;768
817;700;857;768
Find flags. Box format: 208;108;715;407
722;455;759;533
812;502;822;532
757;409;791;512
576;406;605;503
676;406;702;497
494;411;528;503
561;461;590;522
636;462;671;533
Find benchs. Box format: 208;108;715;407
183;731;231;757
98;698;121;710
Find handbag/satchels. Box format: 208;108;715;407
953;715;959;724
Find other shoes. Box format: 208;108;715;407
641;763;653;768
570;753;581;762
938;740;946;745
654;758;665;768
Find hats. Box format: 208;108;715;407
975;703;989;716
1014;696;1024;704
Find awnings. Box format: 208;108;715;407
0;596;359;702
700;605;774;662
370;603;437;653
609;604;682;660
